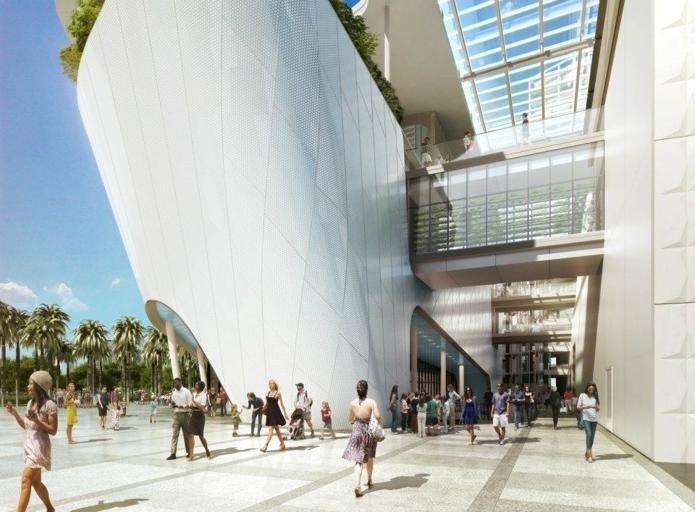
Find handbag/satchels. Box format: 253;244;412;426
368;411;384;441
578;415;585;430
262;403;268;414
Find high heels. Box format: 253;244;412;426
355;480;373;496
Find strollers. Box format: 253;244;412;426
282;407;305;440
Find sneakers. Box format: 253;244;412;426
167;449;214;461
586;453;593;463
497;437;505;445
311;430;337;440
233;432;260;437
261;444;286;452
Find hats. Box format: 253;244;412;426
30;370;52;393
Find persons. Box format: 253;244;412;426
577;383;600;463
421;137;432;167
7;370;58;512
342;379;381;496
387;381;574;446
463;129;472;152
51;377;336;462
521;113;530;145
